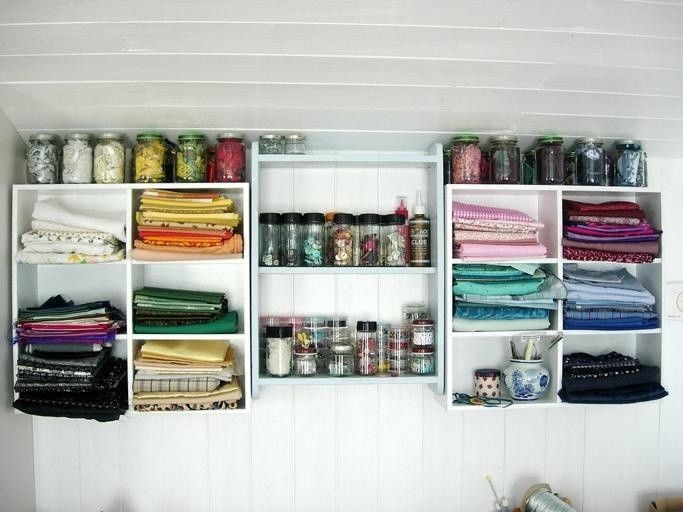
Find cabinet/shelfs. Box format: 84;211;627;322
440;182;668;414
248;139;446;403
8;177;249;423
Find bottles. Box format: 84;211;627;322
257;303;436;378
409;190;431;267
256;214;406;267
283;134;307;155
259;134;281;154
444;137;649;184
25;132;247;184
394;195;410;266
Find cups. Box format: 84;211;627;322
474;369;500;403
